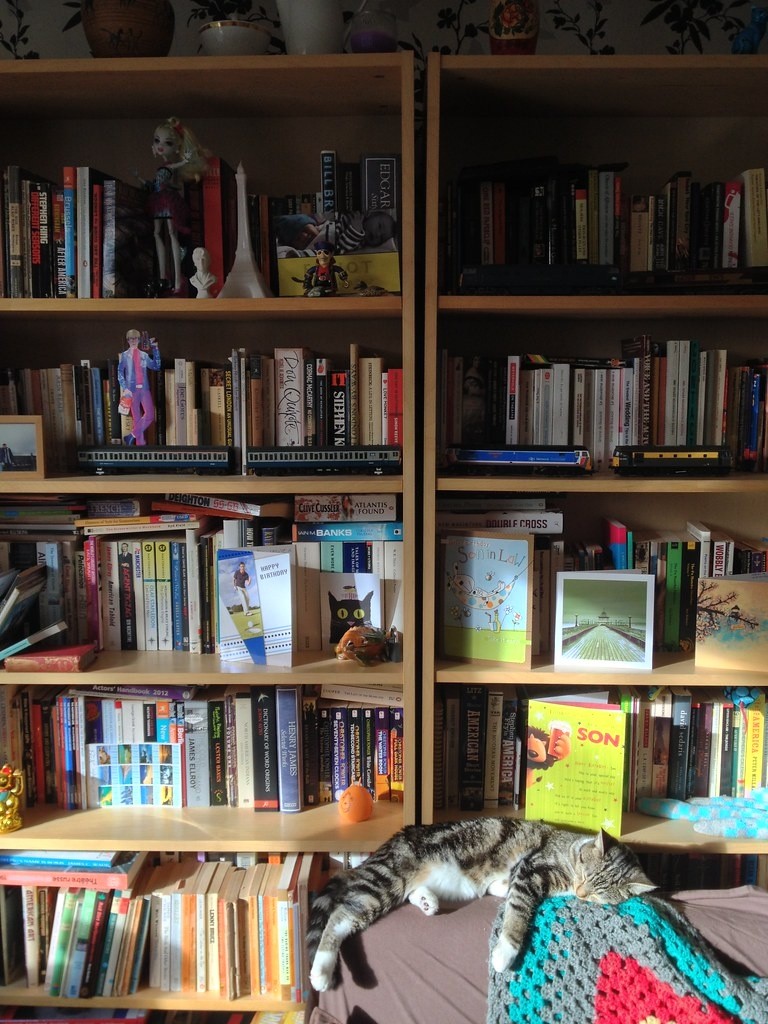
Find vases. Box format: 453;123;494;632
487;0;540;55
79;0;174;56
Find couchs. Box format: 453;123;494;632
305;884;766;1023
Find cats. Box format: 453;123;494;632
307;819;659;992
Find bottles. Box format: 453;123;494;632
274;0;344;55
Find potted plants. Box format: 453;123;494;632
187;0;286;52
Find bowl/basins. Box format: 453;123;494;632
192;19;272;56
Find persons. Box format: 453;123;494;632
277;210;326;257
232;561;254;616
189;247;217;299
119;543;131;568
133;113;207;297
336;210;395;253
0;443;14;471
301;240;349;297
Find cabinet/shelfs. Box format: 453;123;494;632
422;55;768;862
1;52;416;1010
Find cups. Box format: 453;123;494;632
351;9;397;52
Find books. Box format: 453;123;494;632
0;494;767;673
0;851;378;1024
638;852;767;893
0;148;768;299
0;684;766;841
1;340;768;474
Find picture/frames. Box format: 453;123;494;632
0;415;46;479
